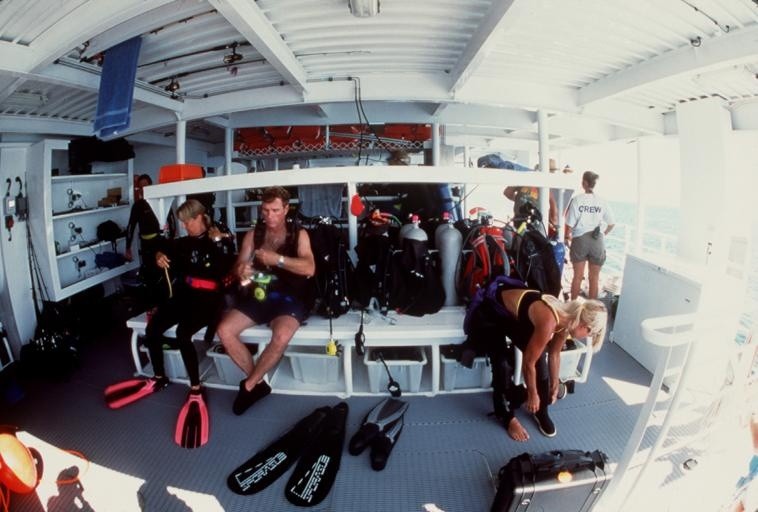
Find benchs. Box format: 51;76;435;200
127;309;594;400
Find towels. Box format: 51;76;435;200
92;35;142;138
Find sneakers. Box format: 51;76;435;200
532;406;556;437
557;383;567;400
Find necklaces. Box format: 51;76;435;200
269;238;279;250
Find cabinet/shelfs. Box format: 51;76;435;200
223;124;451;261
24;138;142;302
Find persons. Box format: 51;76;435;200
504;158;556;238
216;188;316;414
146;199;235;392
455;274;608;441
126;174;176;262
565;172;615;300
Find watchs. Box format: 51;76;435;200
277;256;284;270
212;236;223;242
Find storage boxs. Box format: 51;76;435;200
139;342;191;381
440;351;494;393
280;346;346;384
204;343;258;386
361;346;430;394
549;338;589;385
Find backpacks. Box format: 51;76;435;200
309;225;446;318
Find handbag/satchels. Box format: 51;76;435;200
95;251;130;268
96;220;121;254
490;450;613;512
